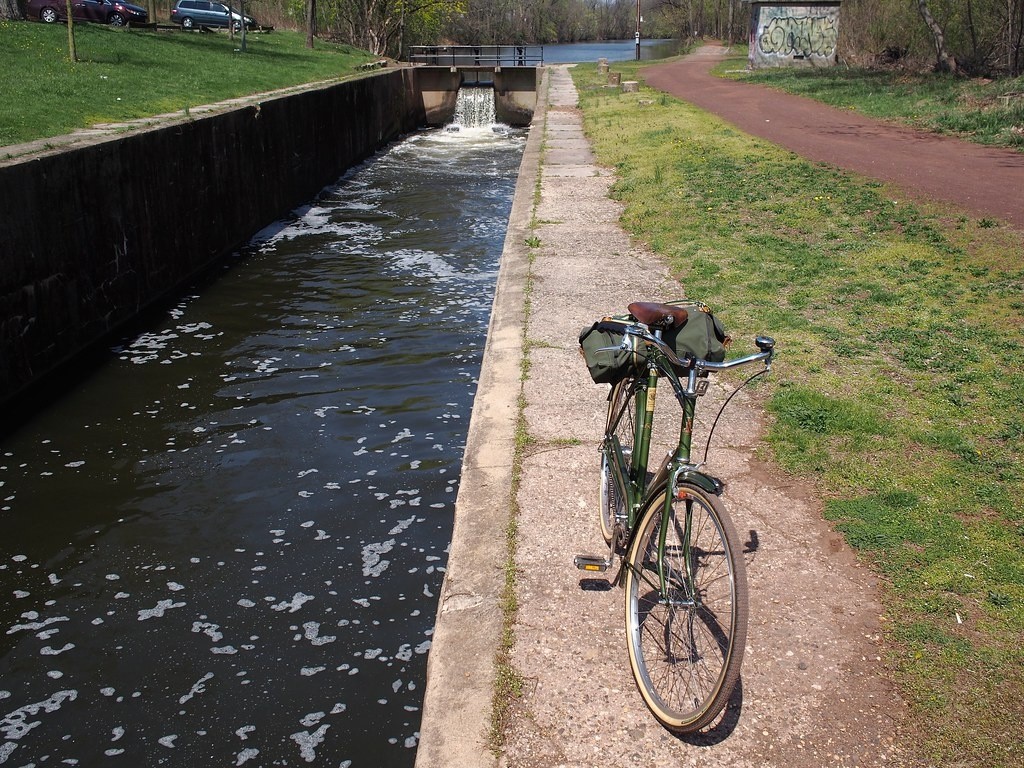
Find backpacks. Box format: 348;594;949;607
578;299;732;384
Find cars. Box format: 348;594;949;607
26;0;148;27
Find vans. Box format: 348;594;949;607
172;0;257;32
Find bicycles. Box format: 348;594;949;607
575;299;777;737
